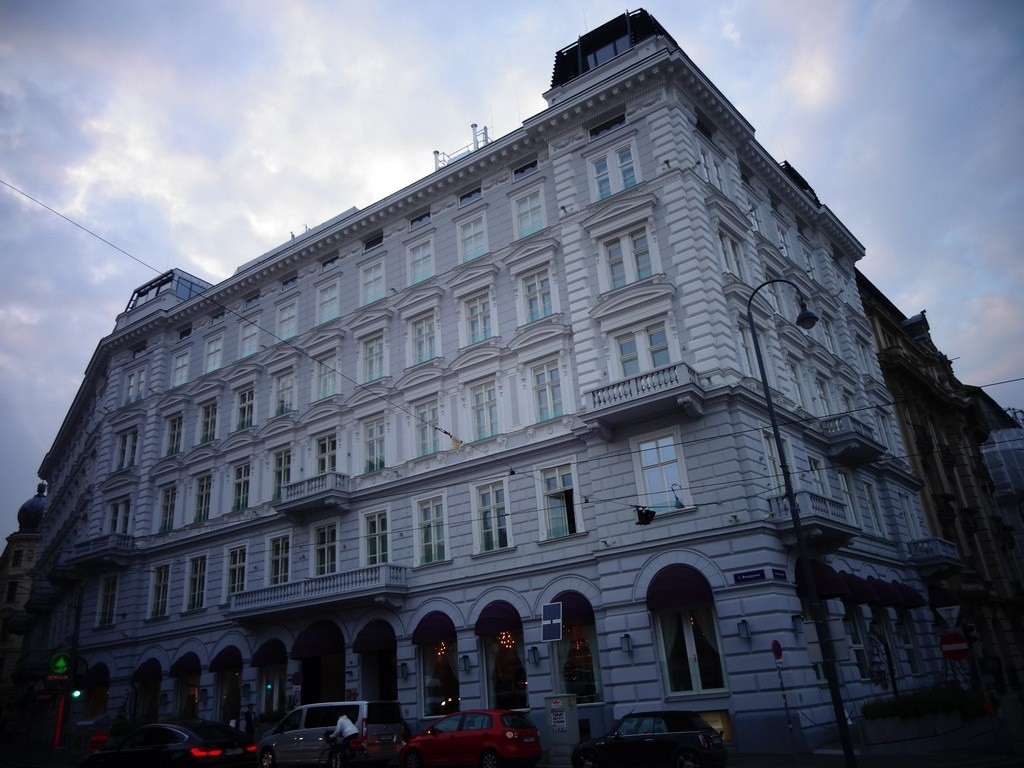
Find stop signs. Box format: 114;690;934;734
940;631;971;662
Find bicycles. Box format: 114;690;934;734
317;728;371;768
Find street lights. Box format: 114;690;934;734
746;278;861;768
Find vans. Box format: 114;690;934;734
256;700;406;768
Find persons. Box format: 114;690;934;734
245;703;257;736
327;711;359;761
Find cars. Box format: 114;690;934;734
570;711;729;768
78;719;259;768
398;709;545;768
